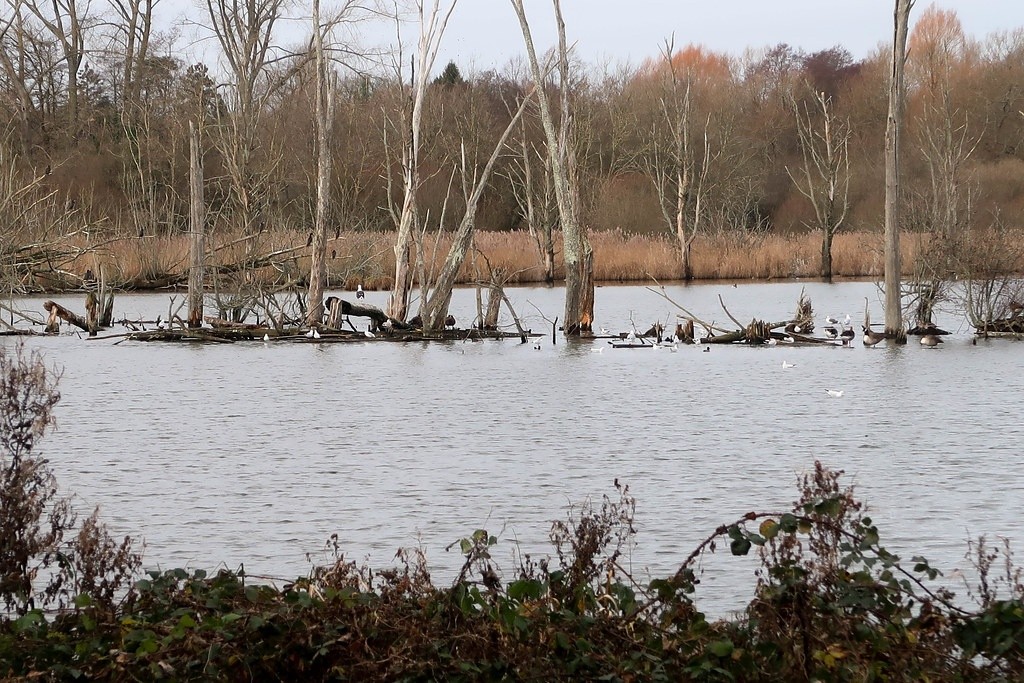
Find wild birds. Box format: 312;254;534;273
0;165;1024;397
